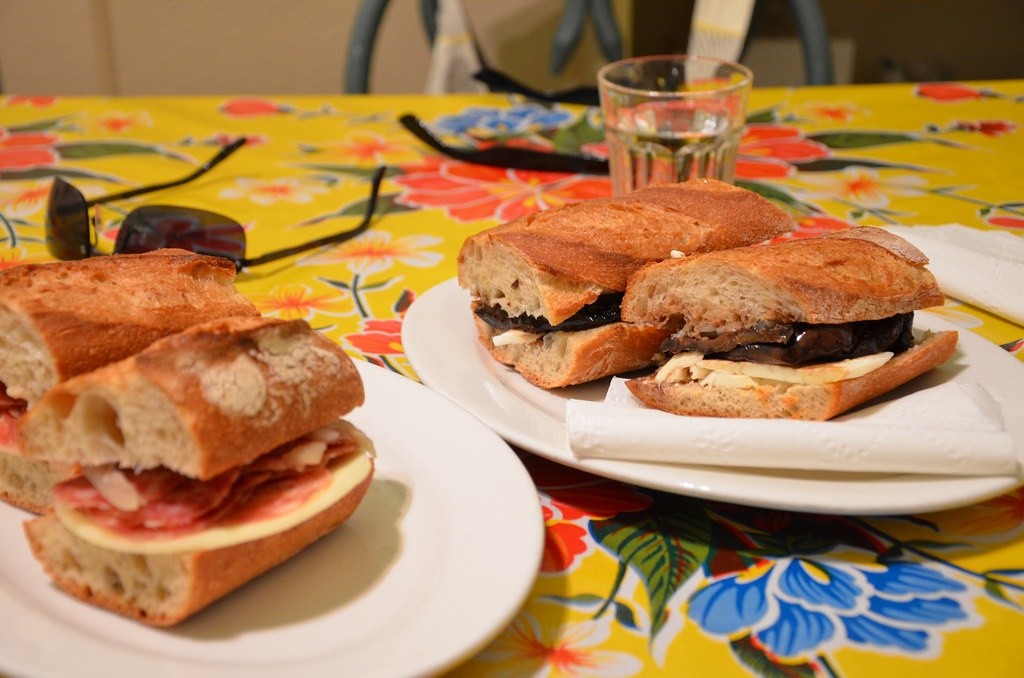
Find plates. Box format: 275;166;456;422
0;357;546;678
401;277;1024;518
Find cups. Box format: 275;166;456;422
596;53;754;198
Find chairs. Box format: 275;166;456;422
345;0;830;92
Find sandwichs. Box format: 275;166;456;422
0;248;378;625
458;178;796;391
621;224;958;421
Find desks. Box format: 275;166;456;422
1;80;1023;676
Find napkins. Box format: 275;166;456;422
881;224;1024;328
